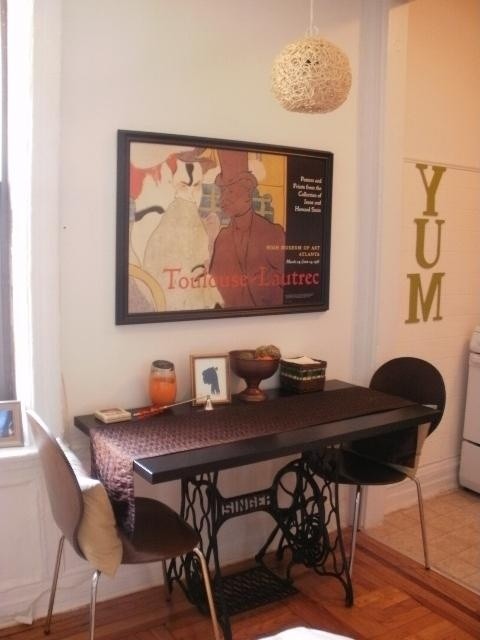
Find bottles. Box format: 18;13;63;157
149;359;177;407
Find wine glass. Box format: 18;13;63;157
229;349;280;402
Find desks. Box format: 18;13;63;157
71;378;444;639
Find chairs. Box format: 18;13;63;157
24;407;223;640
276;355;447;602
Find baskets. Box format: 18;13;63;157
279;356;327;394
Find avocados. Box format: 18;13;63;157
239;346;280;360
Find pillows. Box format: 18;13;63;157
54;436;124;581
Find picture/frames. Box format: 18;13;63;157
114;129;334;327
0;400;24;449
190;354;233;408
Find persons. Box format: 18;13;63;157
127;140;229;313
210;147;286;311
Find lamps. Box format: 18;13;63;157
273;0;353;114
